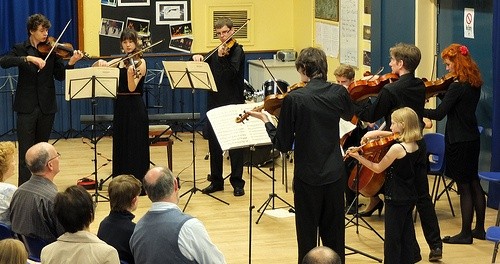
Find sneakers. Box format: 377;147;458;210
234;188;245;196
201;182;224;194
346;204;356;215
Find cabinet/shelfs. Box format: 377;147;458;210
247;59;301;92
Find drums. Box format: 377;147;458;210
243;145;280;167
262;80;289;98
244;78;255;102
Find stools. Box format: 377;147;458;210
149;124;173;170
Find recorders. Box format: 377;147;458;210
276;49;298;61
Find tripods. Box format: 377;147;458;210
345;159;385;242
53;66;120;203
149;61;295;224
0;72;20;148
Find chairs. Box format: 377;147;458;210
425;125;500;263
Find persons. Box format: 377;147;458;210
334;66;433;216
40;185;120;264
302;246;341;264
247;47;355;264
0;15;83;187
189;18;245;197
97;175;142;264
129;166;227;264
0;239;28;264
8;141;66;257
92;24;150;195
346;107;423;264
422;44;486;245
0;141;18;227
354;45;442;261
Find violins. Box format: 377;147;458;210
422;73;458;99
124;51;143;79
344;131;401;160
222;38;237;55
38;36;89;61
348;73;399;101
235;83;306;123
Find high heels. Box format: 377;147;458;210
359;199;384;216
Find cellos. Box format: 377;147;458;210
344;120;393;216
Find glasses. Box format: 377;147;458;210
215;30;230;36
46;153;61;167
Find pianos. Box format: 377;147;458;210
79;113;201;191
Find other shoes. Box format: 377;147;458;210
429;247;443;262
472;229;486;240
442;236;474;244
